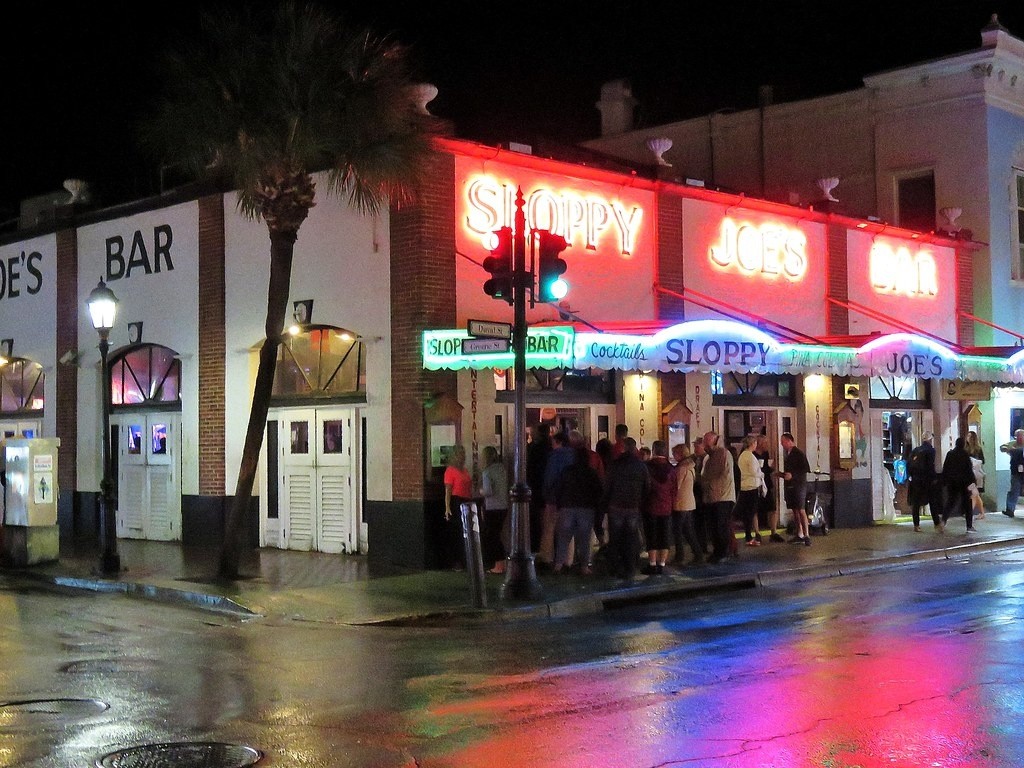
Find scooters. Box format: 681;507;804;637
804;469;832;536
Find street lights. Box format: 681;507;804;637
85;275;123;573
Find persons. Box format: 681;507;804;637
940;438;979;533
1000;429;1024;517
736;436;764;546
153;438;166;454
130;437;140;454
906;431;944;533
444;445;472;572
963;431;985;519
478;446;508;573
523;422;741;579
781;433;812;546
754;434;785;542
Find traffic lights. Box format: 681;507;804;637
538;229;571;302
482;226;513;303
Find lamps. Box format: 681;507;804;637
60;350;81;369
946;382;956;395
289;312;303;335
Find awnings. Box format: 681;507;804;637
421;317;1024;387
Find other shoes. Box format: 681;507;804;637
640;564;666;575
490;560;507;574
746;538;761;547
755;534;761;541
1002;509;1014;517
976;513;985;520
941;521;948;527
666;559;682;566
452;563;465;572
770;534;785;543
594;546;608;558
551;564;563;574
966;527;978;533
707;555;727;563
579;567;592;576
913;526;921;531
935;524;944;533
640;551;649;558
788;536;812;546
688;556;704;565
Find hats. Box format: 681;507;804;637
692;437;704;444
922;431;934;441
